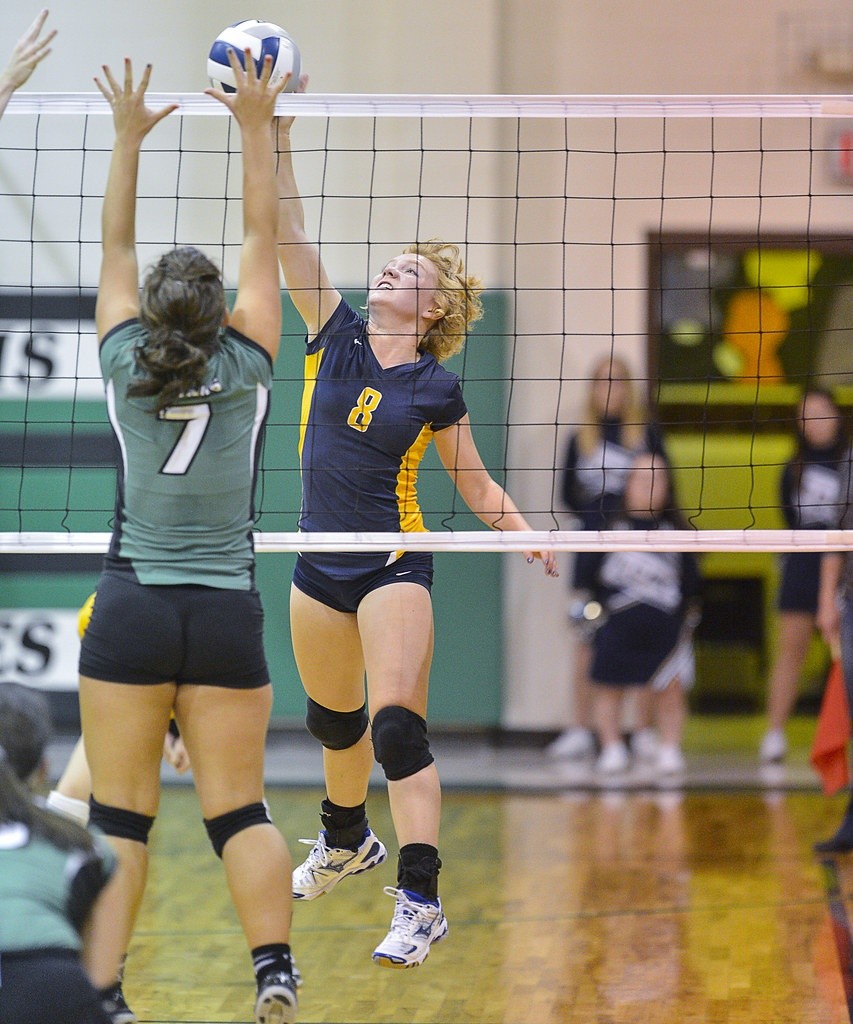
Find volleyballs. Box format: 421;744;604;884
205;18;303;95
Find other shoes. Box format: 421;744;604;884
759;731;789;761
543;727;598;758
653;746;684;775
629;728;659;760
814;824;853;851
594;743;629;775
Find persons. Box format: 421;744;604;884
541;360;703;776
276;71;563;972
1;677;114;1024
1;7;56;122
814;447;853;855
73;46;295;1024
757;386;851;764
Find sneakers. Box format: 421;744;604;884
253;968;299;1024
289;829;389;903
102;981;138;1024
290;956;304;989
371;886;450;970
